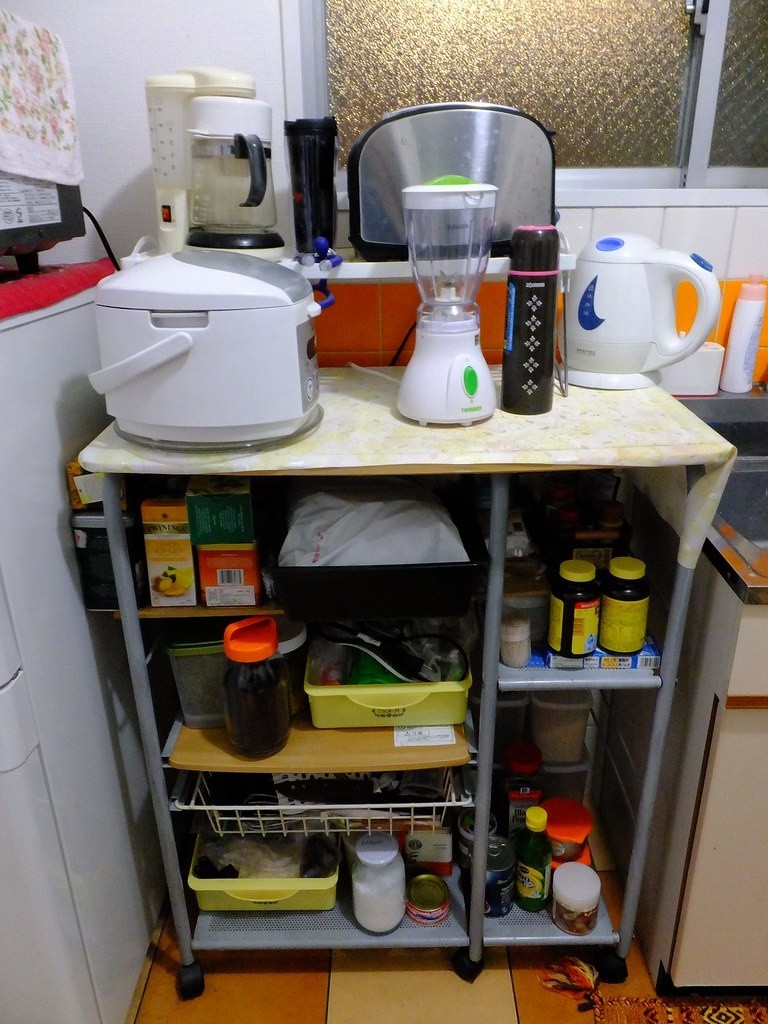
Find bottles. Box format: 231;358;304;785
347;742;604;936
222;615;291;761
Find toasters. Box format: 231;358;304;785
348;102;561;264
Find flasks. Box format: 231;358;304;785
499;224;559;415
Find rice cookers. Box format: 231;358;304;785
87;251;324;448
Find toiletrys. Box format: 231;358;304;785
716;269;768;394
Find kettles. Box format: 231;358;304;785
554;233;723;389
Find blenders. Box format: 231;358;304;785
145;66;256;264
395;175;496;427
283;115;338;257
186;96;289;265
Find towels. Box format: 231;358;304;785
0;5;92;189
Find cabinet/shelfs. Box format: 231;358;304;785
79;360;737;992
664;693;768;988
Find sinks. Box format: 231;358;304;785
683;425;768;583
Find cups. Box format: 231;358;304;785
501;480;653;674
